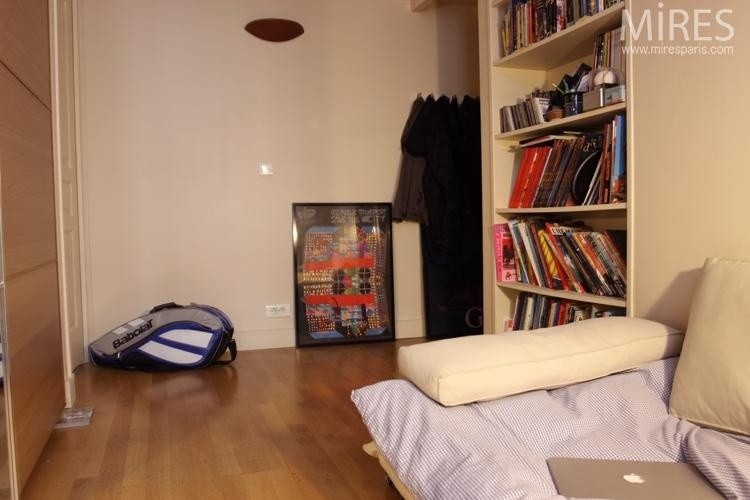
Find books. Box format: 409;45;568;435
493;0;626;334
55;405;94;428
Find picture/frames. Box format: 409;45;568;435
290;202;396;350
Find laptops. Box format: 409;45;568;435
546;457;727;500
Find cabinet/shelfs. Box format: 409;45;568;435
478;0;635;333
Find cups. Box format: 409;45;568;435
563;91;587;118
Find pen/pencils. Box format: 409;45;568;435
552;78;575;94
